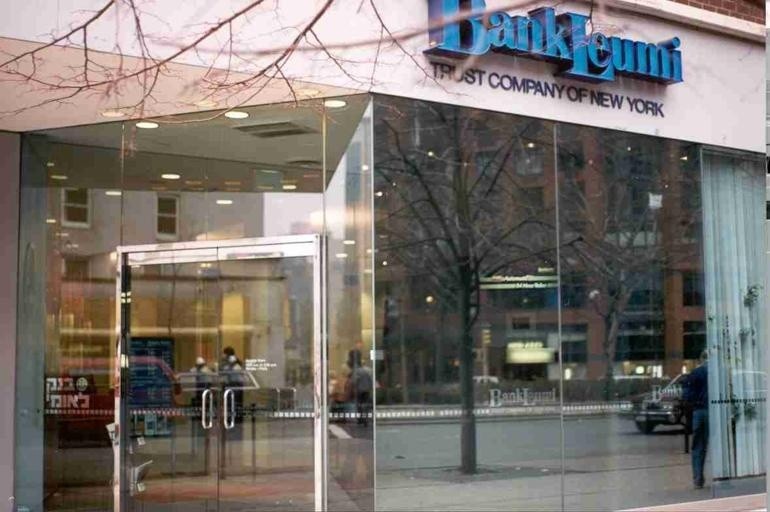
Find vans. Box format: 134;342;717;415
46;351;183;442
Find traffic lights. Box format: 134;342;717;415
482;329;491;345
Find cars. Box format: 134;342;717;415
630;370;691;436
472;375;500;383
172;369;299;413
726;369;770;419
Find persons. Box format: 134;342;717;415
684;350;709;489
334;348;374;488
224;356;242;371
224;345;241;366
189;354;212;375
327;369;337;395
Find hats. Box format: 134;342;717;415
196;356;204;366
228;355;237;362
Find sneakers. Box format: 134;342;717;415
694;477;704;487
